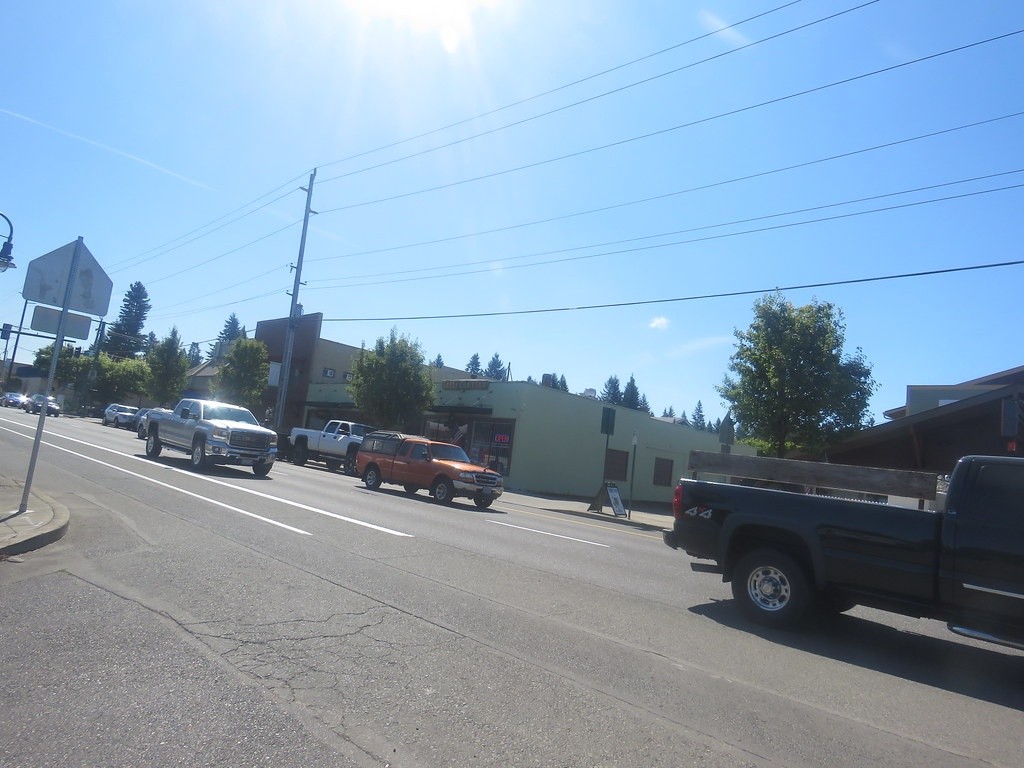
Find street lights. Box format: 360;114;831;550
0;213;17;273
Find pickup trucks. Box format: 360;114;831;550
356;430;504;508
290;420;378;477
146;399;278;476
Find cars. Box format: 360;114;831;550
0;393;61;418
102;404;173;439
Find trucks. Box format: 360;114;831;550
663;449;1023;650
